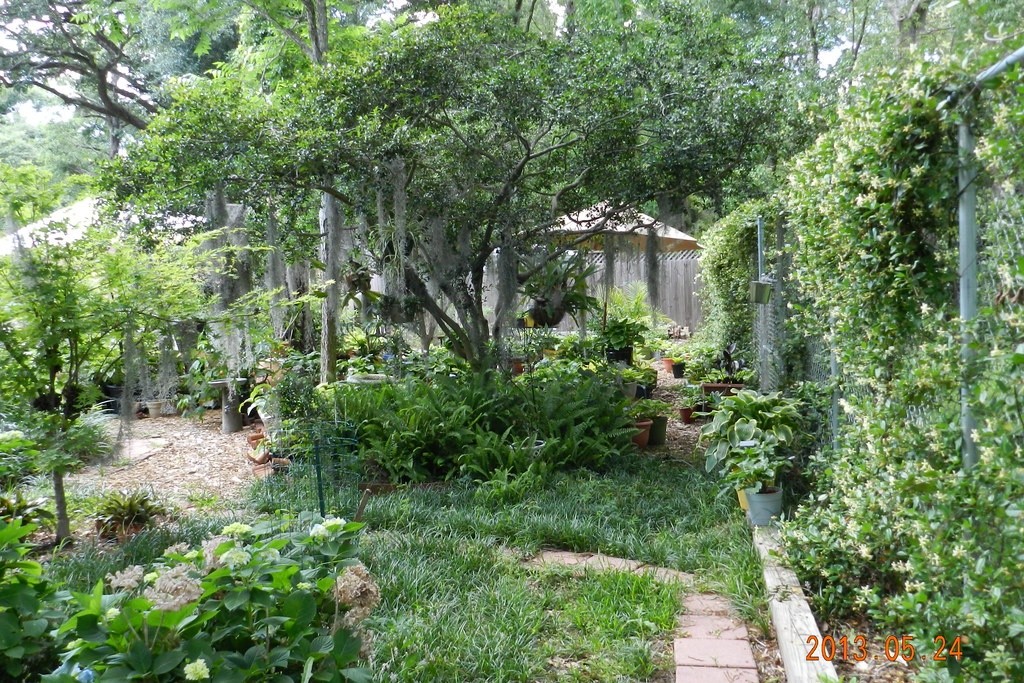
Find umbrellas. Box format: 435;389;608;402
512;195;705;331
0;191;212;356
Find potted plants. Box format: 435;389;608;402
91;244;804;547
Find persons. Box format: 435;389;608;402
529;280;567;330
